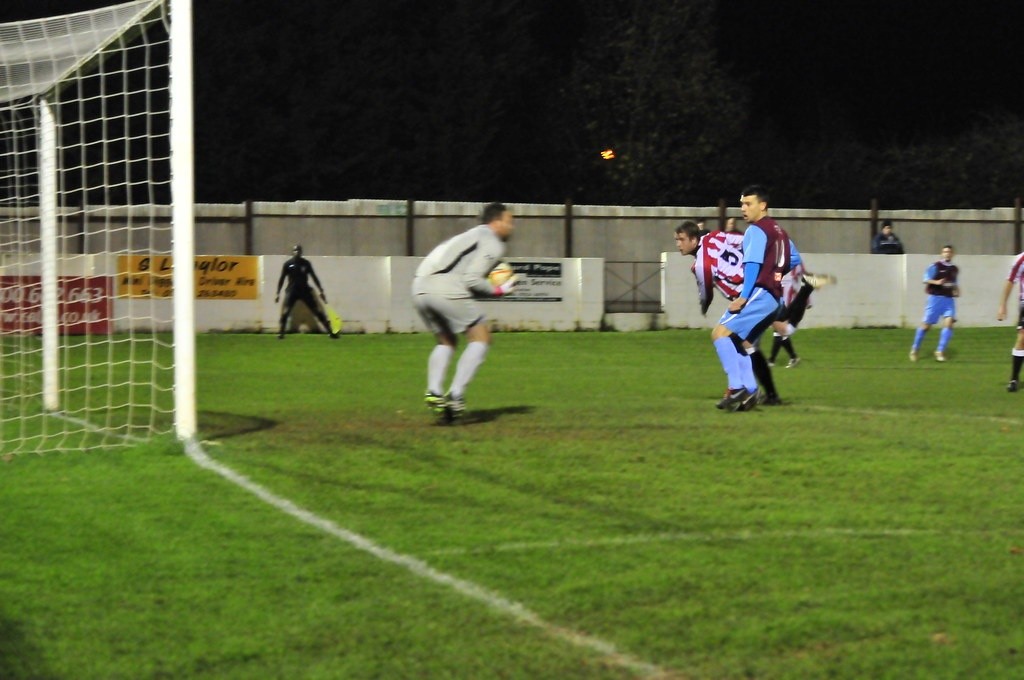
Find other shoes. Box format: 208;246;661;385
767;362;775;368
909;350;917;362
935;350;947;363
785;356;799;368
1007;381;1018;391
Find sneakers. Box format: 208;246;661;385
717;385;750;410
446;387;466;422
735;389;761;413
761;393;782;405
424;391;450;418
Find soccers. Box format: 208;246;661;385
488;262;514;286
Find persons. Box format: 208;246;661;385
871;220;905;253
997;252;1024;390
674;185;834;411
909;246;959;361
412;204;519;423
275;245;338;339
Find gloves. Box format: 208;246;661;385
500;275;519;294
